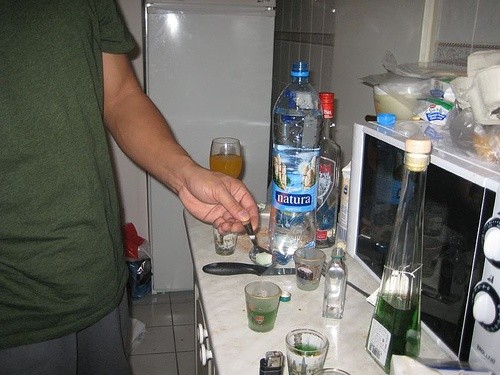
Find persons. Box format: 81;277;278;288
0;0;258;375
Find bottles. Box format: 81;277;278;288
271;61;324;266
315;91;343;249
364;137;432;374
323;247;348;320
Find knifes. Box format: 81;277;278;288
203;262;295;276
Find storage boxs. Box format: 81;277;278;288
125;257;154;303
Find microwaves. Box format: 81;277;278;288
340;120;499;375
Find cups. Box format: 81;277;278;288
245;280;282;332
292;247;326;292
211;227;239;255
285;328;349;375
209;136;243;179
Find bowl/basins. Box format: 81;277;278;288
372;92;420;122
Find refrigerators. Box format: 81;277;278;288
109;1;279;295
